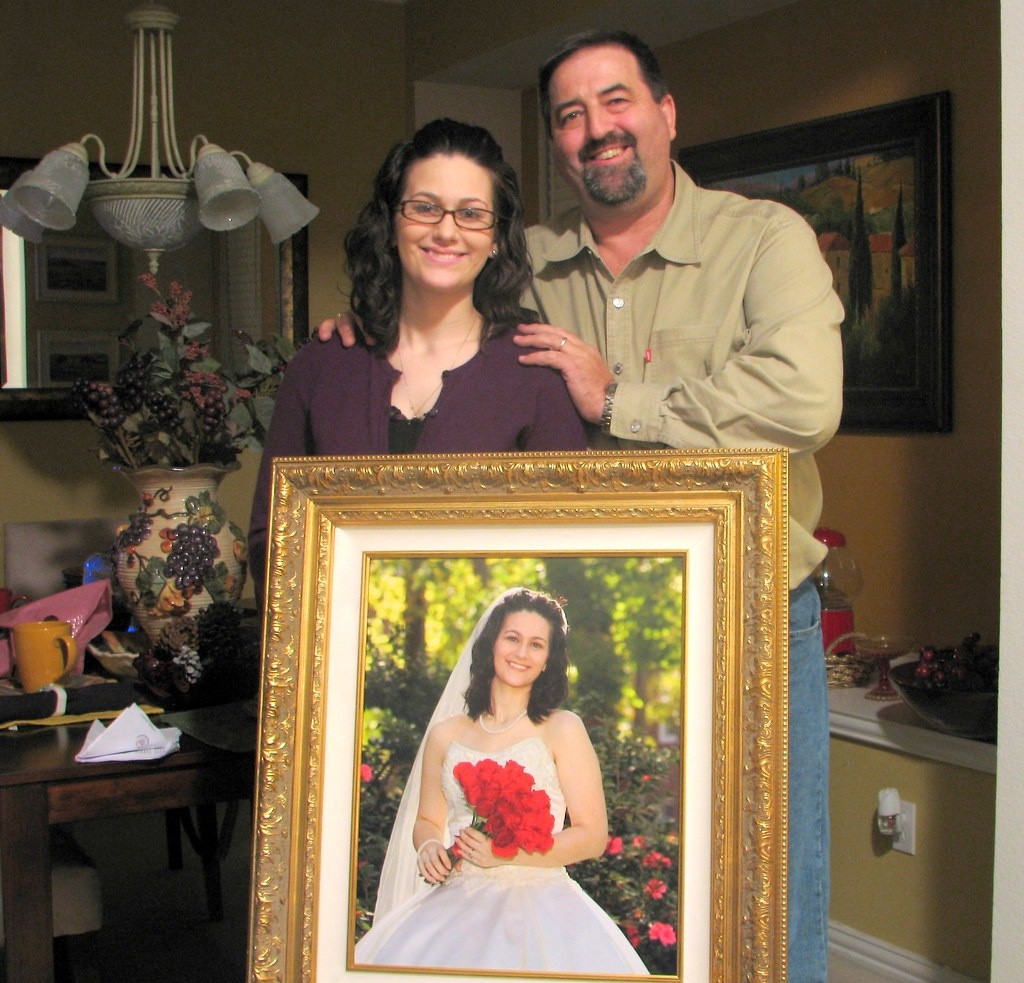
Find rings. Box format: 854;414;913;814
335;311;344;320
559;337;567;351
469;850;473;857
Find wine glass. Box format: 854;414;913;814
855;633;915;702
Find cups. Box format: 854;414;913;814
12;621;77;694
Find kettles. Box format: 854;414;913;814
806;526;864;659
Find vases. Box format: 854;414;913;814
109;461;249;648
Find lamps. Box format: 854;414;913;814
0;0;320;275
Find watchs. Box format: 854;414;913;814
599;381;618;435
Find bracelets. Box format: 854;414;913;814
417;839;443;855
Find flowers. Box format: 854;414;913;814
71;274;324;470
418;757;556;886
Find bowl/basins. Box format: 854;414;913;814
885;658;999;744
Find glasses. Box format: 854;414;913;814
397;200;498;230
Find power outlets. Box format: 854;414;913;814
893;801;916;856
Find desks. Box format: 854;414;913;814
0;677;257;983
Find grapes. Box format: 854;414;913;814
74;367;228;436
913;632;999;696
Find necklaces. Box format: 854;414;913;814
396;314;475;418
480;711;528;735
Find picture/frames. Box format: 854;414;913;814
249;445;792;983
679;88;955;433
35;233;121;305
36;330;121;387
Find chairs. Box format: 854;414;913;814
5;518;136;636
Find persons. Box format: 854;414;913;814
352;587;653;976
246;116;595;626
317;28;844;983
